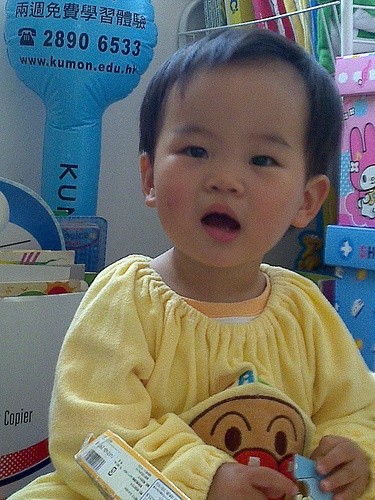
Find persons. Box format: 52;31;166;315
10;26;375;500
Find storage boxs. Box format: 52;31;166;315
331;52;375;230
322;224;374;376
